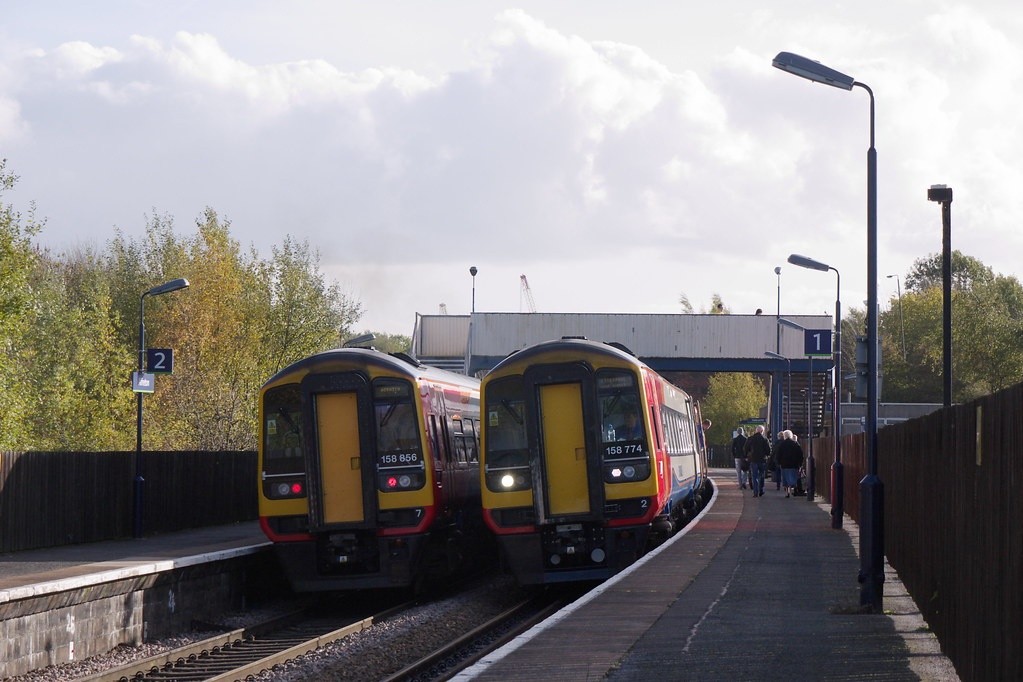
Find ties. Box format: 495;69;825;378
626;428;634;441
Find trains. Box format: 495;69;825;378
480;333;707;577
257;346;485;591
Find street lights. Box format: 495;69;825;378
778;316;817;500
137;277;191;451
787;255;844;535
766;352;792;498
887;274;907;363
772;51;881;616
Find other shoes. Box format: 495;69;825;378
753;491;758;499
739;483;747;490
749;478;753;489
776;485;782;490
759;490;765;498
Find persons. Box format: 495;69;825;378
731;427;749;489
792;433;801;447
697;419;711;448
743;425;771;498
776;430;804;497
770;431;784;490
613;410;643;440
715;303;724;314
755;309;762;315
